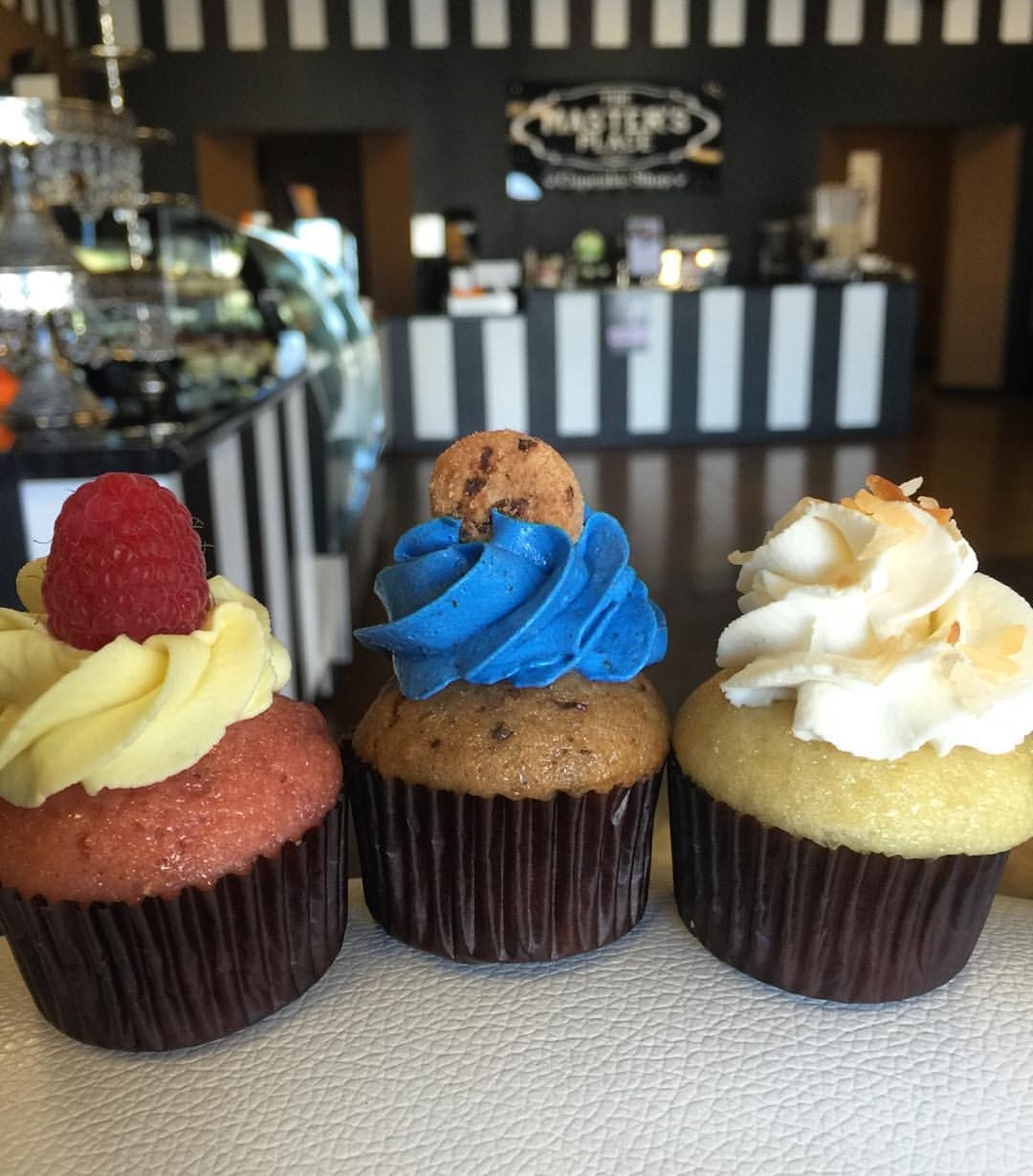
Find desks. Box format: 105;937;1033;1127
1;314;373;703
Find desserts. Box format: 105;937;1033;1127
666;475;1033;1002
0;470;352;1052
338;431;670;966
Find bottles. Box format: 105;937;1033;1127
10;323;82;430
